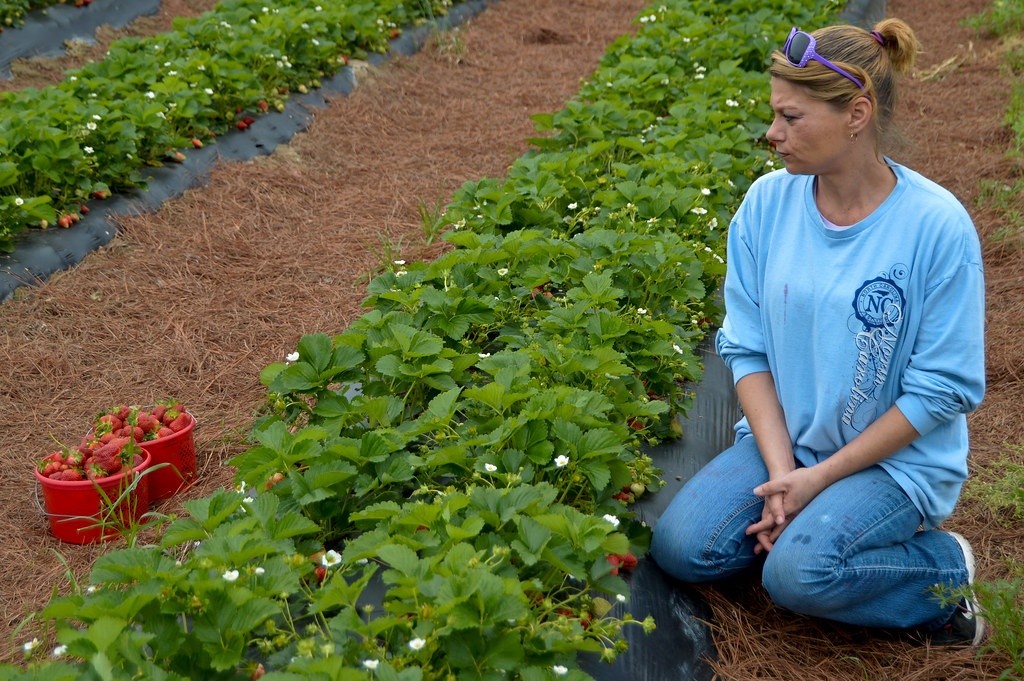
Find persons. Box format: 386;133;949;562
651;18;985;650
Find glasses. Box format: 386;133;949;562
781;27;869;98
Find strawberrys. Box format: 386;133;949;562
555;379;684;629
37;398;192;481
40;29;401;229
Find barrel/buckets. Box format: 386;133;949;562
34;447;151;545
81;405;198;503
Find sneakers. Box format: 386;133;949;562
946;530;983;645
